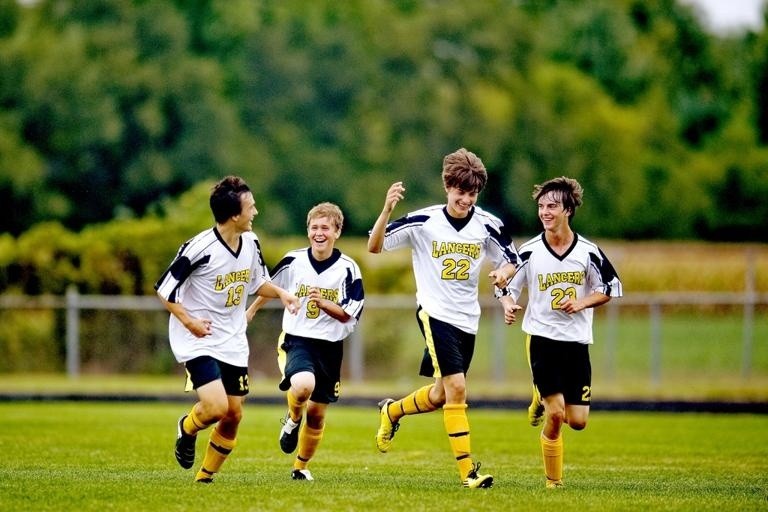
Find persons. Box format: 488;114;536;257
367;148;523;488
495;176;623;488
246;202;364;480
154;175;301;484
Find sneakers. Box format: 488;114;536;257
291;468;314;482
377;397;399;454
279;411;301;453
527;383;546;426
175;415;196;468
462;469;494;489
